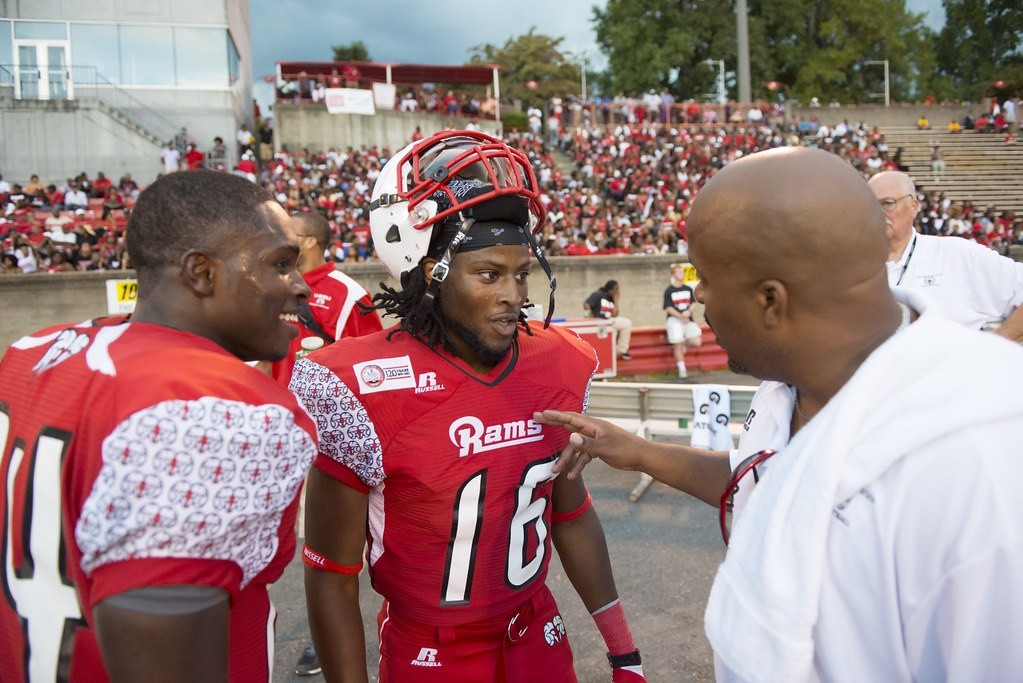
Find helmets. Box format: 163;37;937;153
369;130;546;288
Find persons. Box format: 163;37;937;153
258;214;381;675
0;170;319;683
494;86;817;256
663;265;702;379
533;146;1023;683
160;98;391;262
280;60;360;97
396;89;496;140
867;170;1023;343
0;171;139;273
814;95;1023;245
288;130;646;683
583;280;632;360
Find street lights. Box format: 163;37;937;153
702;58;724;105
864;60;889;107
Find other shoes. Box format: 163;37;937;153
623;353;632;361
678;370;687;379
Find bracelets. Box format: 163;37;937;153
607;648;642;668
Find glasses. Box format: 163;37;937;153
880;194;913;211
720;448;777;545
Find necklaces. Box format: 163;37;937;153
795;302;910;420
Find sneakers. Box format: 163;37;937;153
293;642;323;675
